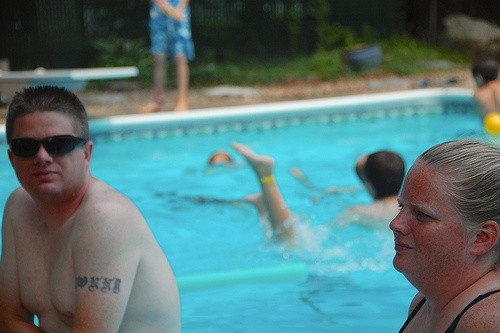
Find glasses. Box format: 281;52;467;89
9;135;92;156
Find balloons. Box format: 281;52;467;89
483;112;500;136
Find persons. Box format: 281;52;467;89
185;140;406;252
472;59;500;120
0;86;180;333
389;140;500;333
138;0;196;112
